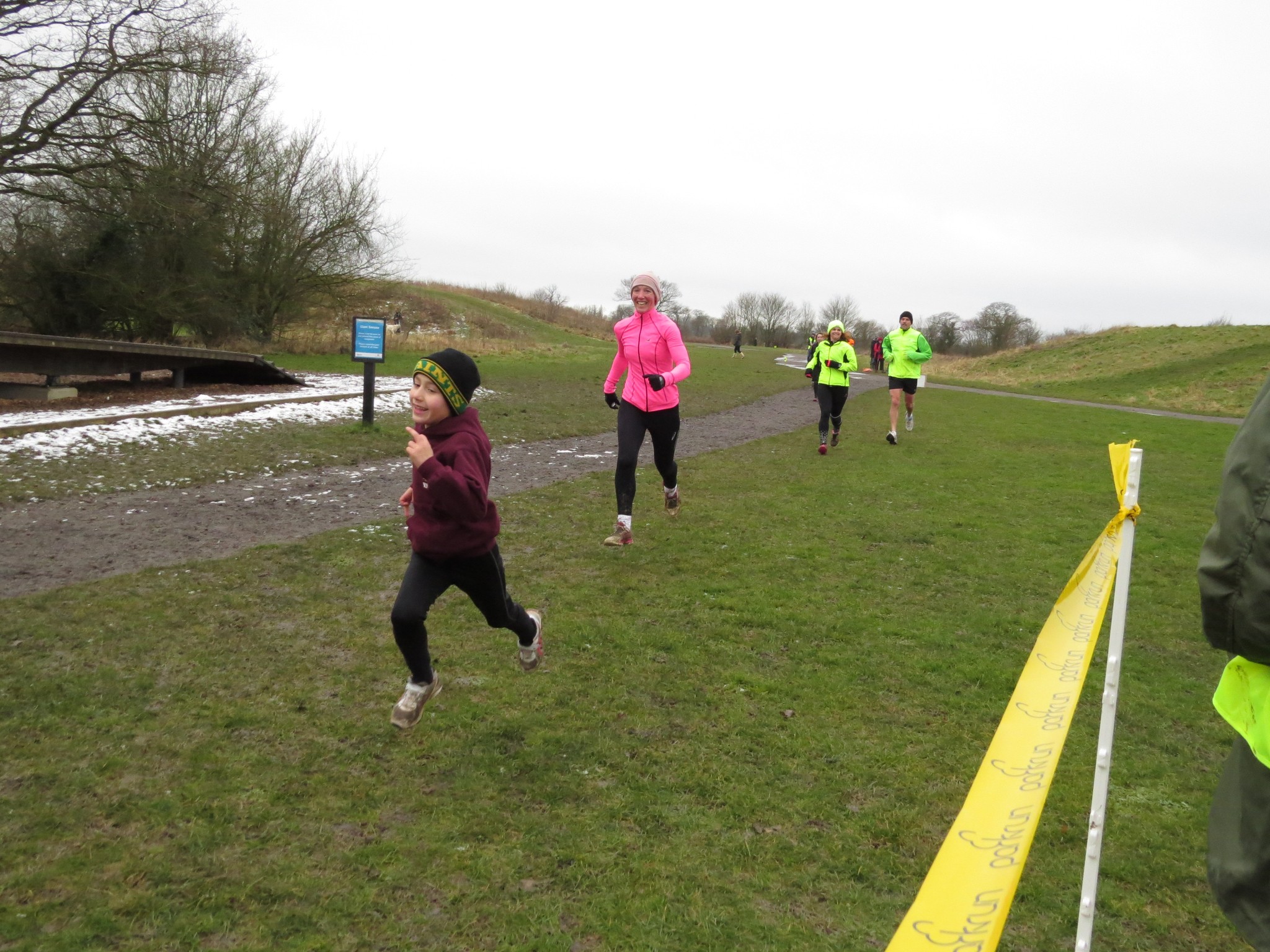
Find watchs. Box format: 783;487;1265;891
838;363;842;368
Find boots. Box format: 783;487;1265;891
731;353;737;358
740;352;745;359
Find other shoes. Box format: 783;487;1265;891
811;381;814;387
813;398;818;401
881;370;886;373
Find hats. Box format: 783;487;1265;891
735;330;739;333
411;347;481;416
899;311;913;324
827;320;844;334
630;271;661;305
878;337;883;341
811;332;816;335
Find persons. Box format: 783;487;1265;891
807;332;817;356
602;276;692;549
844;332;854;347
731;330;745;359
804;319;858;454
880;311;932;444
875;337;886;374
869;335;879;369
806;332;825;401
386;346;542;730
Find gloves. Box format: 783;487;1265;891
904;351;911;360
604;392;620;410
642;373;665;392
824;360;841;369
885;353;894;362
805;369;813;378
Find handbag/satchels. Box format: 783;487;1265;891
862;368;872;372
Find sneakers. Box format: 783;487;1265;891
604;522;634;546
830;430;840;446
390;667;443;729
905;411;913;431
818;445;827;455
517;608;543;671
662;479;680;517
886;431;898;445
